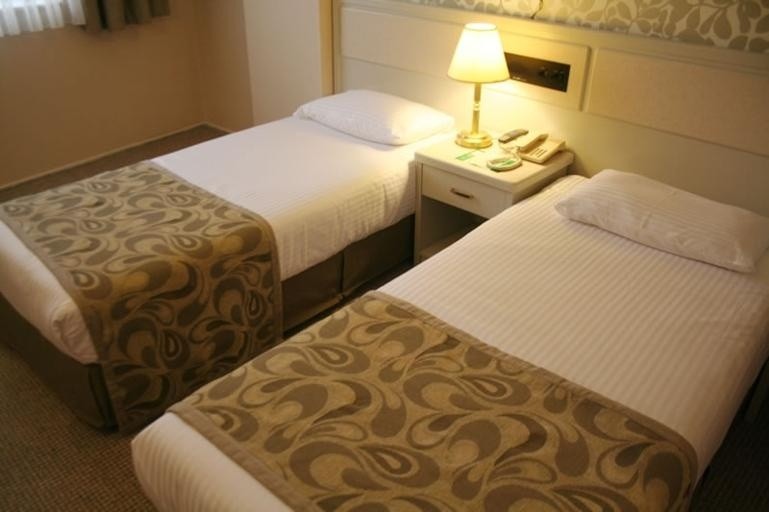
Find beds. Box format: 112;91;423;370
0;113;450;433
130;176;769;512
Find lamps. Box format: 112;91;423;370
446;23;513;148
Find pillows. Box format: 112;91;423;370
293;89;454;146
552;169;769;274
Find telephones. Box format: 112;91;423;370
514;131;566;164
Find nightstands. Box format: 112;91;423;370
415;134;576;266
